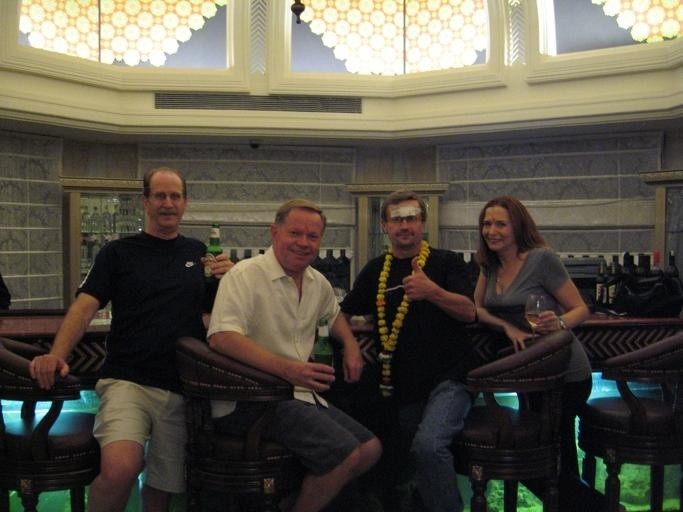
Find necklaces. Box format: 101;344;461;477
374;238;431;399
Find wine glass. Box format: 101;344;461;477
524;294;552;342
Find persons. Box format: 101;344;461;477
29;166;234;512
204;197;384;511
473;193;629;512
335;188;477;511
0;271;13;512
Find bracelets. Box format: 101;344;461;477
557;315;567;329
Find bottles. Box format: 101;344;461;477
594;250;679;308
203;224;265;282
80;205;119;231
80;240;110;270
312;249;350;290
310;318;335;383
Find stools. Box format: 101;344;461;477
175;338;305;512
575;331;682;511
451;326;573;512
1;336;105;511
